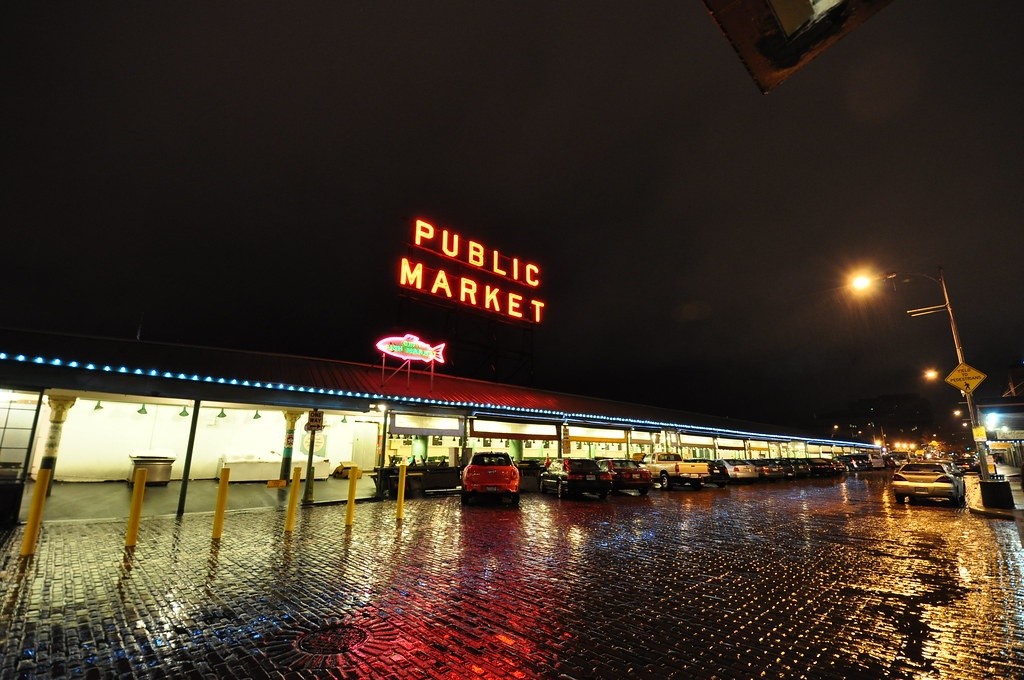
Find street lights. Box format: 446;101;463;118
923;369;983;451
851;271;990;482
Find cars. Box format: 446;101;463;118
747;452;979;507
713;459;759;484
539;458;613;500
595;459;654;495
461;451;521;506
684;457;731;489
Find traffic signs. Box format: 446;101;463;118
308;411;323;430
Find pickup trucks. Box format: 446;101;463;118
638;452;711;491
